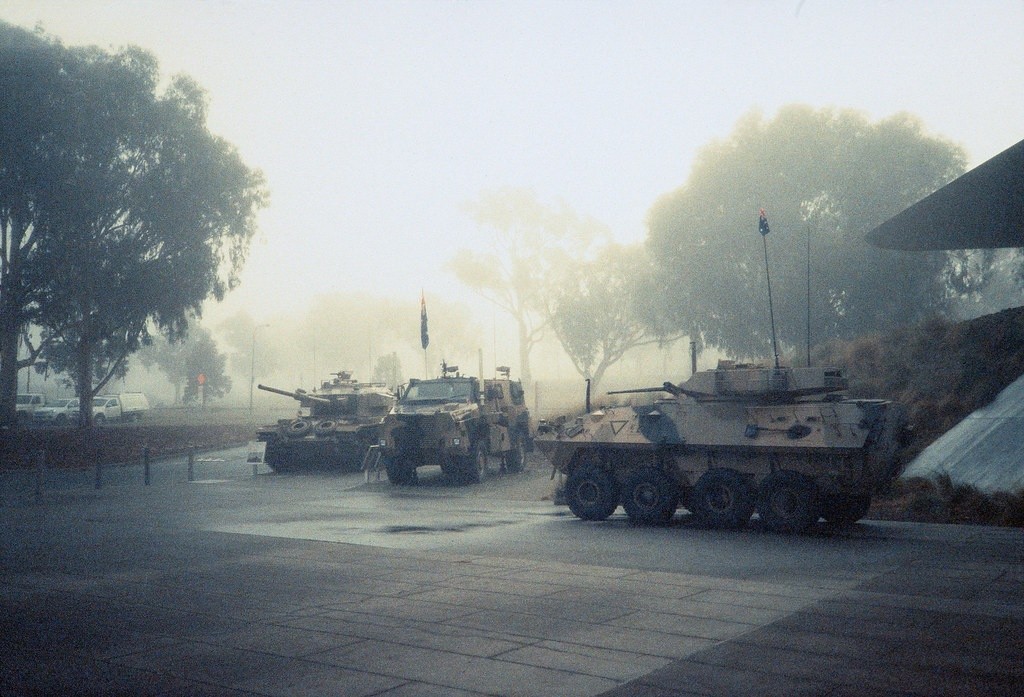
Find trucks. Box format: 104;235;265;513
64;391;149;429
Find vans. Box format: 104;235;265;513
15;394;46;420
36;398;80;425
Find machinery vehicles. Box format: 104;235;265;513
374;367;534;486
254;378;397;474
534;211;909;536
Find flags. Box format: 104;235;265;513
421;289;429;349
759;207;771;235
